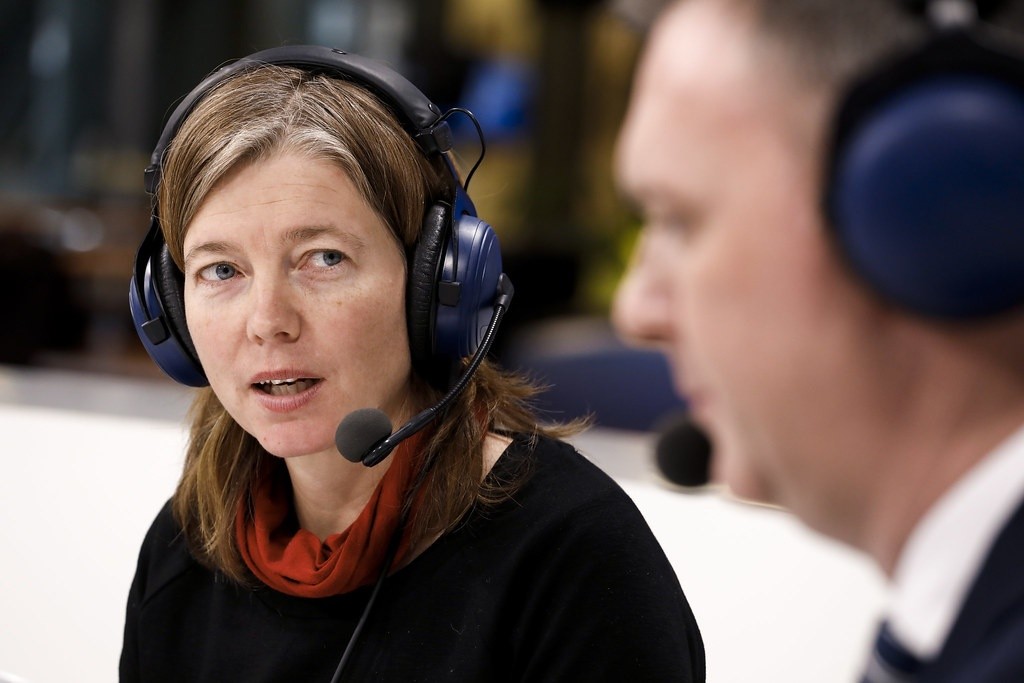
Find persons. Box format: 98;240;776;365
601;1;1024;683
103;44;712;683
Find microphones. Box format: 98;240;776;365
652;419;713;489
335;306;505;467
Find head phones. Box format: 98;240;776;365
814;1;1024;321
127;43;515;389
